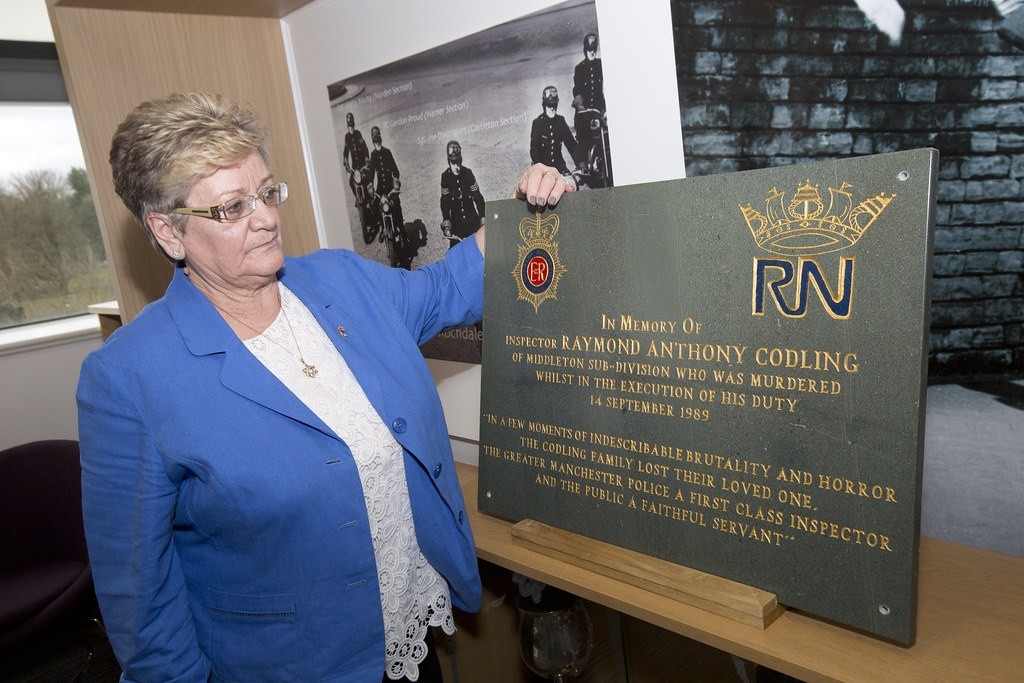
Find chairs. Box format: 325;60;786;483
0;438;112;683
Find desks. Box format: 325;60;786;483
430;464;1024;683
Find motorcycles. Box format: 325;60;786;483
443;226;481;241
347;163;403;269
562;109;612;191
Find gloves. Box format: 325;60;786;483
393;178;401;191
480;217;485;226
440;220;452;237
367;183;373;194
576;163;588;174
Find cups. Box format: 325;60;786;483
518;598;593;683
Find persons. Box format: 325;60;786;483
363;125;409;249
75;93;576;683
341;109;374;203
440;140;487;251
529;85;589;178
572;33;606;172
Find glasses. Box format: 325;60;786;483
157;181;289;220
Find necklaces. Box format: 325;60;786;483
210;279;318;378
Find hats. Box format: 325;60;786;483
543;86;558;104
584;34;598;49
447;141;461;160
347;113;355;127
372;126;380;142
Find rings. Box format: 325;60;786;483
543;171;554;177
